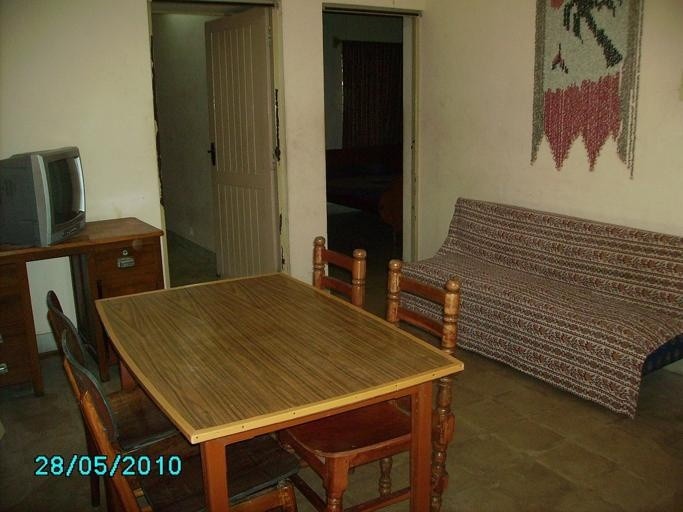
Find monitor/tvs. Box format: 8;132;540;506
0;145;88;248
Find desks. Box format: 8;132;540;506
93;269;464;512
0;217;166;397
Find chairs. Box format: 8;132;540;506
58;361;305;512
251;237;369;436
44;288;221;512
279;257;463;510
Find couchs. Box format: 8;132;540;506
399;198;683;420
